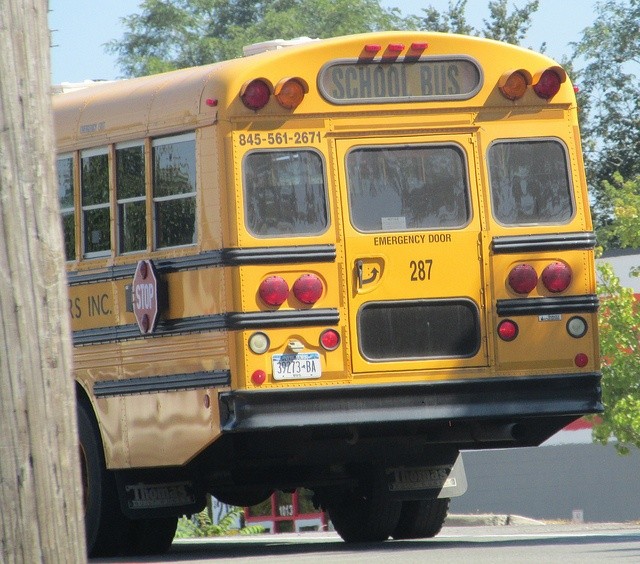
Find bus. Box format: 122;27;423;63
52;30;609;556
